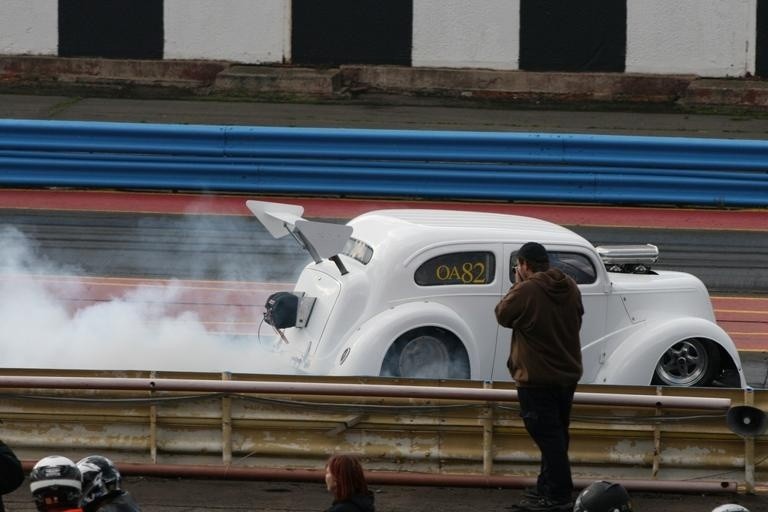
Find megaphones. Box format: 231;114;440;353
726;401;768;436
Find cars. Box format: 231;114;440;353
234;193;751;389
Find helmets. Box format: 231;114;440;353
75;455;121;501
28;454;80;499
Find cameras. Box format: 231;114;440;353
512;265;518;273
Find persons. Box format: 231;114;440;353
322;455;375;512
0;441;26;512
494;242;585;510
76;455;141;512
29;455;85;512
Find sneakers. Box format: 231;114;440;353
520;486;574;511
